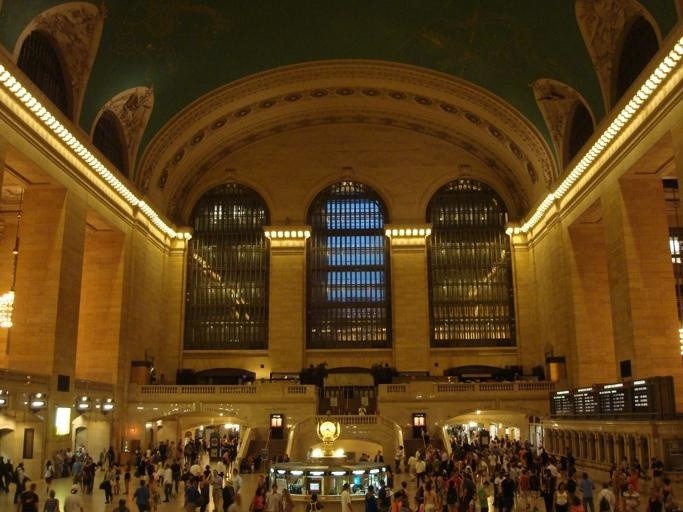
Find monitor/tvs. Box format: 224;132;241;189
290;484;302;494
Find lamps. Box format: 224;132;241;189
0;387;115;415
0;185;25;328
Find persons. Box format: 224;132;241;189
0;405;679;512
